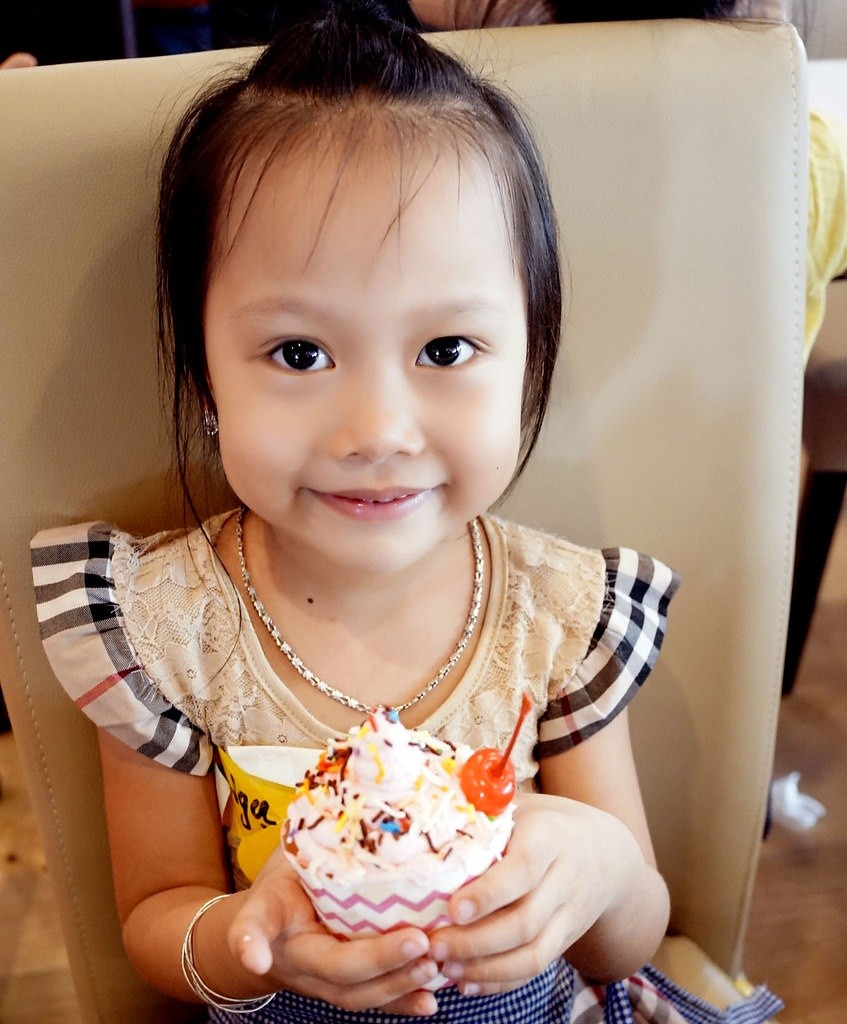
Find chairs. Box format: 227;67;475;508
0;15;812;1024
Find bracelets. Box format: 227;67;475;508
180;892;276;1013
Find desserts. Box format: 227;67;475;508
281;692;533;992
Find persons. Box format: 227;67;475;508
409;0;846;843
25;28;786;1024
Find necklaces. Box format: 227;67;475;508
237;506;484;724
